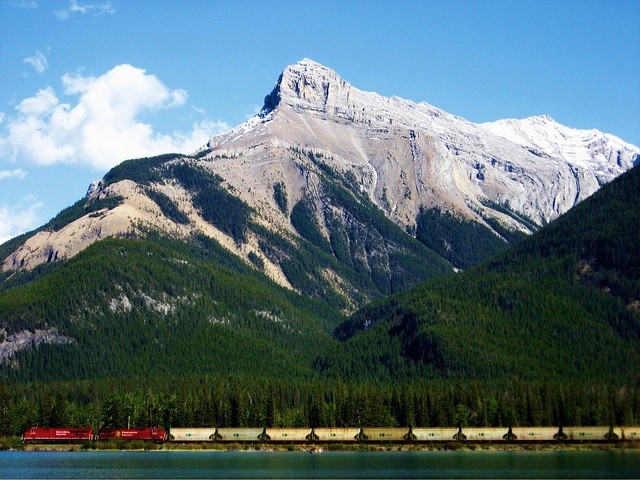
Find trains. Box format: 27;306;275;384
21;425;639;446
